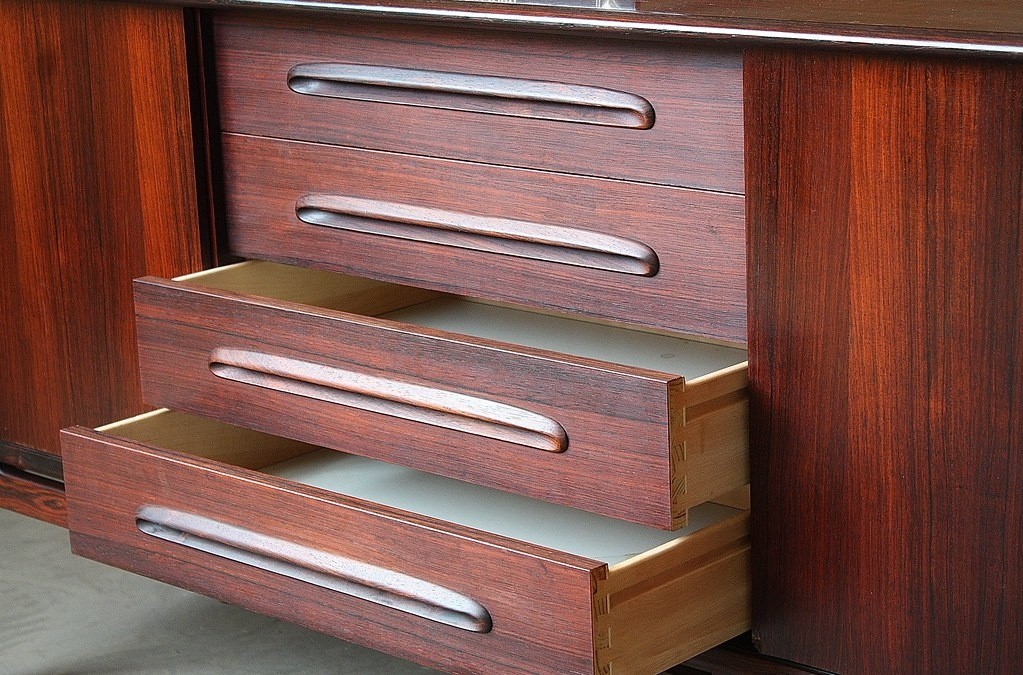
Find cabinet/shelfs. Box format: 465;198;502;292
1;0;1023;675
